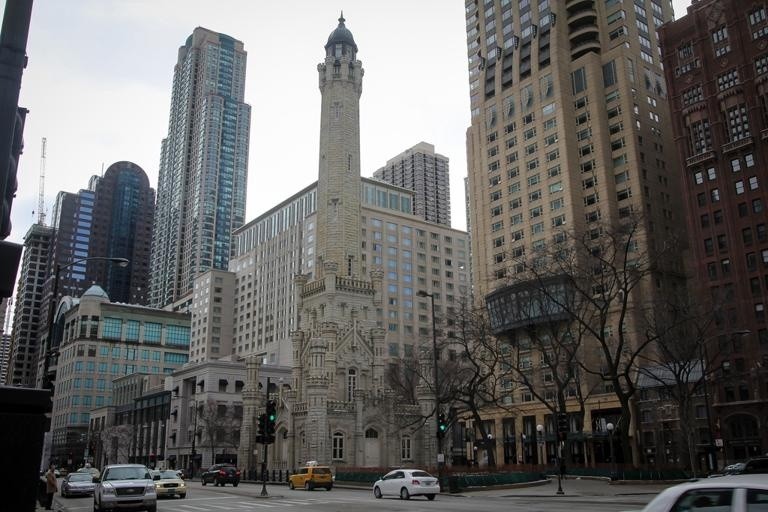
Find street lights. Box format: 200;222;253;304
42;257;129;389
606;423;615;481
696;331;752;471
416;290;443;492
537;425;543;464
173;396;198;454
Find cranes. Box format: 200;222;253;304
32;137;47;226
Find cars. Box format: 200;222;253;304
288;461;333;491
373;469;440;500
61;463;186;512
45;469;60;478
201;463;241;488
724;458;768;474
642;474;767;512
180;469;192;480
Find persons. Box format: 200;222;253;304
44;462;57;510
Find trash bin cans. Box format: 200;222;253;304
449;479;457;493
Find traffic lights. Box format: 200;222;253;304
439;413;447;433
257;414;267;435
267;404;276;433
